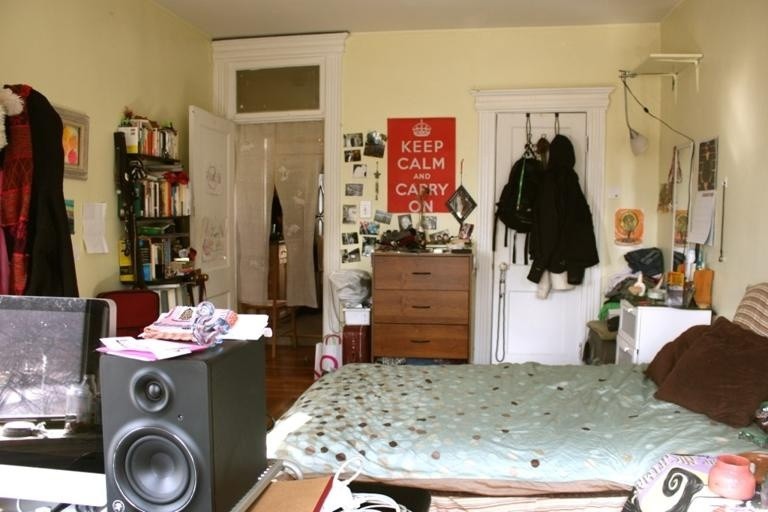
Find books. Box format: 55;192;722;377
685;189;716;247
118;115;192;279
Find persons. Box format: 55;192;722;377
398;216;411;231
698;142;714;191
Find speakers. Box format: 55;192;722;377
99;335;266;512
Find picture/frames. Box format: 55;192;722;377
51;104;89;181
445;185;477;224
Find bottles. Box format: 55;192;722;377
633;271;646;298
708;454;756;501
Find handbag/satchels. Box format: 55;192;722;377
314;335;343;380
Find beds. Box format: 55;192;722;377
266;284;767;497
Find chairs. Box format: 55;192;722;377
241;240;298;359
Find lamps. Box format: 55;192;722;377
616;69;679;157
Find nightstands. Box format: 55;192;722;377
614;298;711;367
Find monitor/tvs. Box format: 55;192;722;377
0;295;117;508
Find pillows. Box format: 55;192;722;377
641;316;767;429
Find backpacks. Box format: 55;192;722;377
493;147;543;265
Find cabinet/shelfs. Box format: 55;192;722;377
370;249;471;365
113;130;200;290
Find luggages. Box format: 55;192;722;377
342;325;371;364
